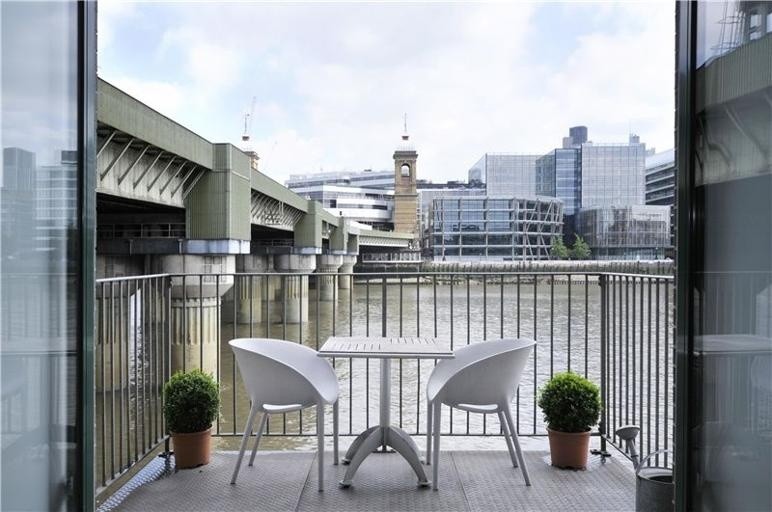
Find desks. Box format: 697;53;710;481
315;335;457;489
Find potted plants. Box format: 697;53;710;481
161;367;222;469
537;371;602;471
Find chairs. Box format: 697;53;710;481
226;336;341;493
424;336;533;491
